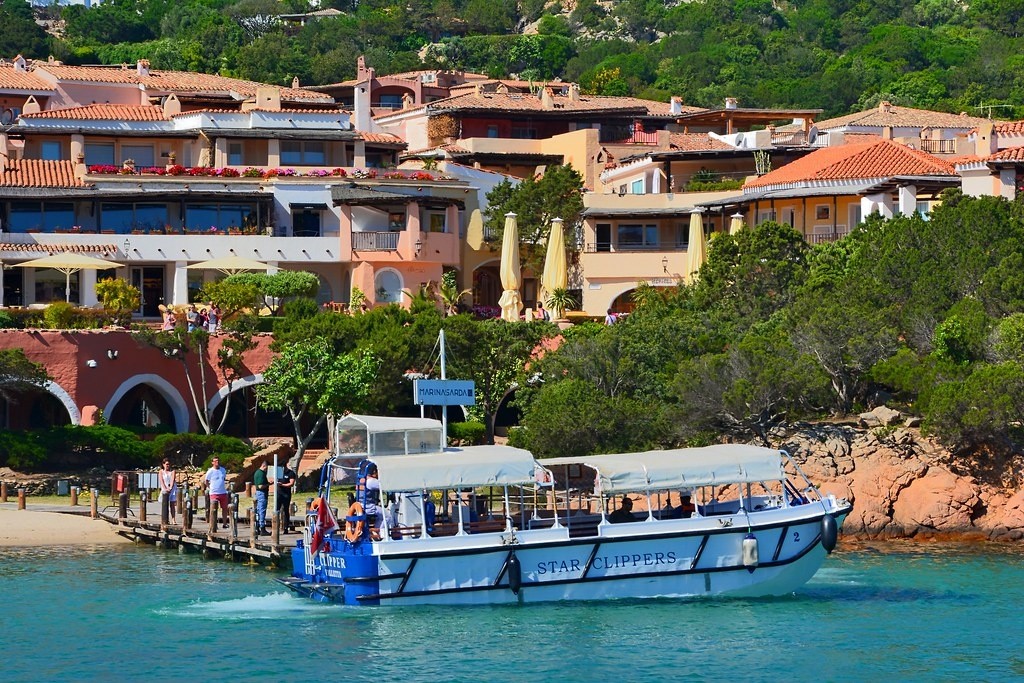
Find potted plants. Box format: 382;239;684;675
168;150;176;164
438;176;460;181
77;153;84;163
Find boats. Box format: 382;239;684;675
274;327;855;608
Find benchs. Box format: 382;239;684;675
370;509;673;542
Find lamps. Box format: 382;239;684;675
662;256;668;272
124;239;130;258
414;239;422;258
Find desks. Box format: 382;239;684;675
333;303;346;314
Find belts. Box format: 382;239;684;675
256;488;269;492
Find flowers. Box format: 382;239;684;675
473;304;503;318
73;216;258;232
89;164;434;181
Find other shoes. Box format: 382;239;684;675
283;526;288;534
223;524;229;528
172;520;177;524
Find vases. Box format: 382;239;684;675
26;229;40;231
55;230;257;235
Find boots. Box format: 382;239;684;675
255;527;260;535
260;528;271;536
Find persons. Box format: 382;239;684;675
609;498;638;523
356;465;435;541
277;459;296;535
604;309;627;326
163;308;177;331
187;301;230;333
158;458;178;525
360;305;367;314
205;456;229;529
671;491;703;518
253;461;274;536
535;301;546;321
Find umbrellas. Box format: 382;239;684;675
539;217;568;320
684;208;707;285
13;250;125;303
498;212;523;322
730;213;745;245
182;253;285;277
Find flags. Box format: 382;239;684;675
310;495;340;560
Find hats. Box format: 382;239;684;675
679;491;692;497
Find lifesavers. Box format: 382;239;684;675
308;497;322;512
345;502;364;544
